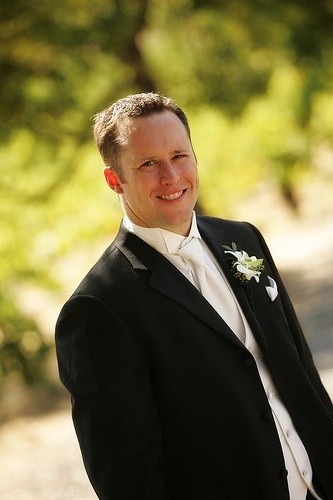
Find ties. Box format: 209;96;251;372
180;237;246;344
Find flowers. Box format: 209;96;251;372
222;241;264;284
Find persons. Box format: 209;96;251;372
55;93;333;500
